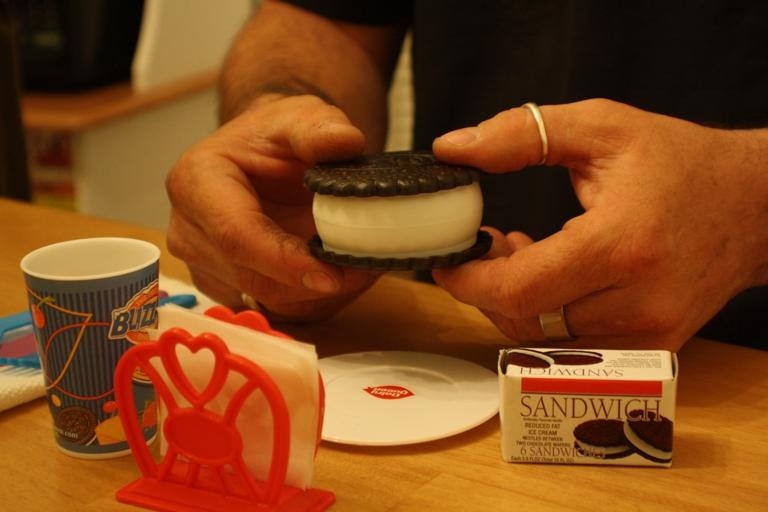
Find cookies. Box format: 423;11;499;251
572;418;635;460
545;350;603;366
622;408;673;464
505;347;554;369
302;149;493;273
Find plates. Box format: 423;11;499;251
317;349;502;448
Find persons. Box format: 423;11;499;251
11;0;145;92
164;1;768;359
0;1;30;201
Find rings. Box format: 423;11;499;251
522;102;550;167
537;307;575;343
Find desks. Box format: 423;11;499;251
0;195;768;512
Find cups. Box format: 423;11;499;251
19;237;161;461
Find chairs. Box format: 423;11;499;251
19;1;255;228
